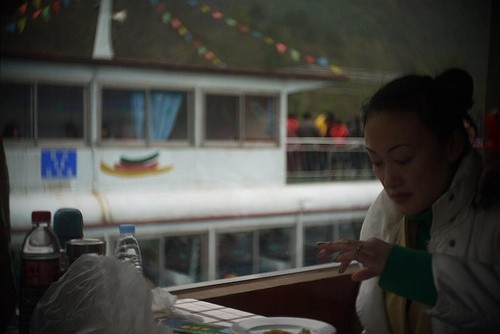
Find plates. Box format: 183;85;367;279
231;316;336;334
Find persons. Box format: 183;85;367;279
288;110;378;179
314;67;500;334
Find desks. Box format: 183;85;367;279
152;298;265;334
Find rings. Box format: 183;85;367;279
357;243;363;251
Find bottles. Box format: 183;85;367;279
53;208;83;251
115;224;143;275
20;211;60;331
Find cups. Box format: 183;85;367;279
66;238;106;269
